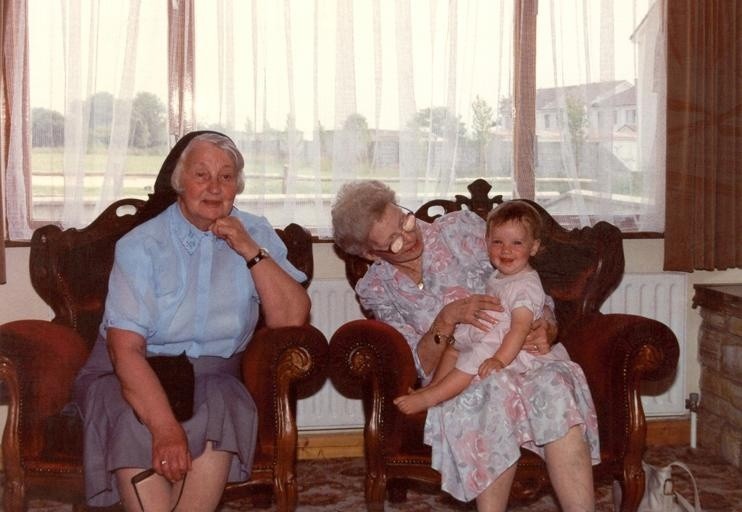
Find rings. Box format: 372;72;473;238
534;344;538;350
475;310;480;316
161;459;168;465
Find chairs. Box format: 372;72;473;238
328;178;679;511
0;199;327;511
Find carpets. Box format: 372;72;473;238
26;443;742;511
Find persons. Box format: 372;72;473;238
75;131;311;512
389;200;549;417
326;179;604;511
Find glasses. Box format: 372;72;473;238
368;201;416;254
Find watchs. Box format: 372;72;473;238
430;323;454;346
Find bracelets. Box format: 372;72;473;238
244;246;270;269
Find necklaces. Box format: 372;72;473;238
415;274;428;291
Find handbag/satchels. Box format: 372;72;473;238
612;458;701;511
